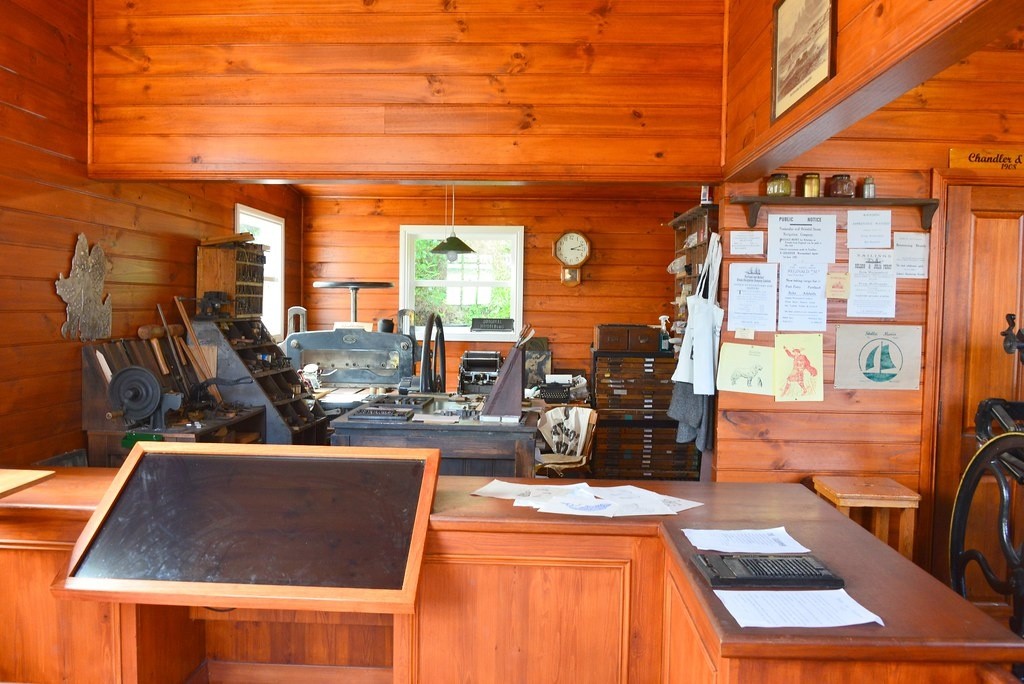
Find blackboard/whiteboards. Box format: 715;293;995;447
49;441;442;615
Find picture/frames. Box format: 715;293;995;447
770;1;836;126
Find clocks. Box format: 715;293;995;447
552;230;592;288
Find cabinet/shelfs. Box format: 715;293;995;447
188;318;328;446
1;469;665;684
668;204;719;360
593;326;660;351
664;480;1024;684
195;245;265;320
591;351;702;481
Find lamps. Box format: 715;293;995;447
429;185;476;262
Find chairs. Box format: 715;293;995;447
536;407;598;478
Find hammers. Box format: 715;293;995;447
137;324;170;376
167;324;187;365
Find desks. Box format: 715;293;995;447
533;399;591;417
330;404;539;479
88;406;267;468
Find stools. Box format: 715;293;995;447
813;476;922;562
